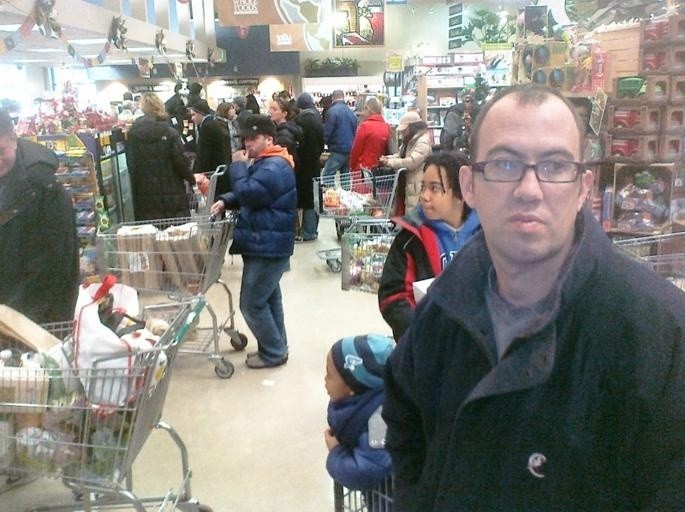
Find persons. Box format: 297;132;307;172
377;111;433;218
323;335;395;489
377;152;484;346
382;87;685;512
322;89;358;191
349;95;389;193
1;108;80;365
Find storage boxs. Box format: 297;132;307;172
118;225;162;290
156;221;209;286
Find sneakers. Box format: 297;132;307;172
247;351;258;357
246;352;289;370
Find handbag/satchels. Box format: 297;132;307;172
74;277;164;409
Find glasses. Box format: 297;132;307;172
471;159;585;183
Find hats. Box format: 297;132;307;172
296;93;314;108
190;99;209;116
233;97;245;108
233;114;276;138
332;334;394;394
395;111;422;132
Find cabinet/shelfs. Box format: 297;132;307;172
36;135;97;283
418;75;511;151
93;125;136;233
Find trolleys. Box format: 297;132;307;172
313;163;407;272
340;219;396;295
96;207;248;379
0;292;211;511
333;466;394;512
191;163;229;214
611;229;684;291
